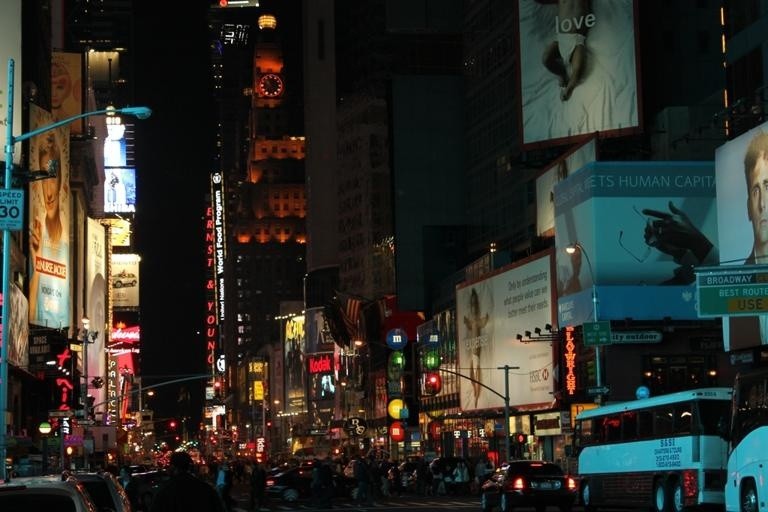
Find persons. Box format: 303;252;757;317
117;445;487;512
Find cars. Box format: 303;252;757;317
263;458;473;504
1;468;167;512
480;460;580;510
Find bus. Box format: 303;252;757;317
573;370;768;510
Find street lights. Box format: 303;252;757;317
516;433;527;445
565;242;606;406
1;59;152;478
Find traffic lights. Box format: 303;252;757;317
214;379;224;401
265;412;273;432
426;373;442;395
168;419;181;446
64;445;75;464
